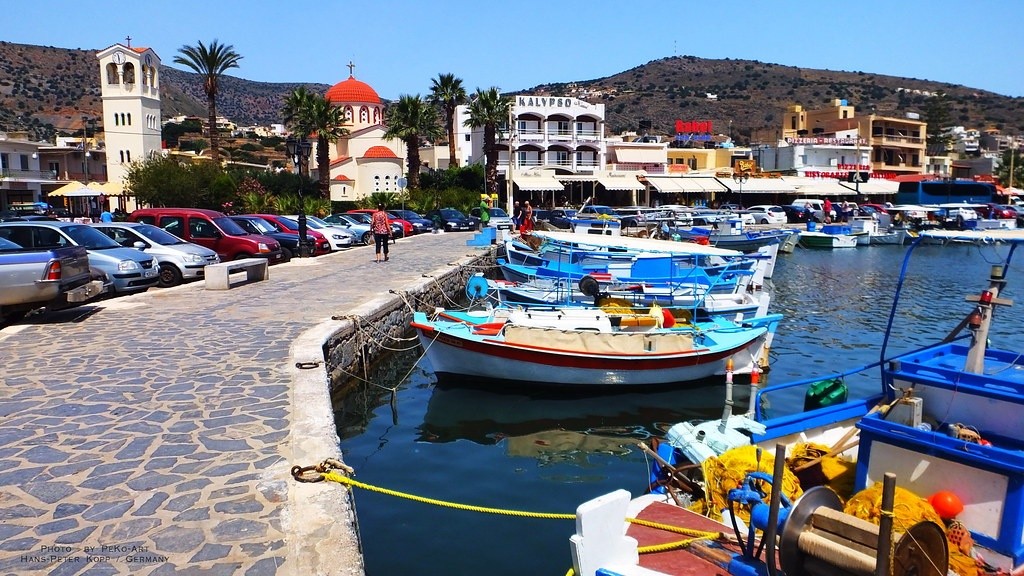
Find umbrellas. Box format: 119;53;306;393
47;180;139;214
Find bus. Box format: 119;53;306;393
896;181;1003;204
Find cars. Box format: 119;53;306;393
164;205;435;257
425;207;475;232
546;197;1024;227
512;210;550;227
467;207;514;231
0;214;219;295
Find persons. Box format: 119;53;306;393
841;196;849;222
370;202;393;264
821;197;833;224
88;138;105;150
100;208;115;223
522;201;533;232
804;201;813;209
510;201;523;234
480;194;492;227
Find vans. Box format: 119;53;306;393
128;209;283;274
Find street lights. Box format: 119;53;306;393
284;135;314;258
732;172;750;218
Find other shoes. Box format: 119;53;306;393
384;257;389;261
511;232;516;234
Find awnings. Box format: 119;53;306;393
839;177;900;194
781;177;858;195
715;178;796;193
512;173;564;191
645;177;728;193
594;170;648;190
614;147;668;164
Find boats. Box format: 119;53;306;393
421;375;754;447
566;233;1024;576
408;220;919;391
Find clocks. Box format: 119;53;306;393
145;54;153;68
113;50;126;65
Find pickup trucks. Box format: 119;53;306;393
0;246;104;310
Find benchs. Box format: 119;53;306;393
204;257;269;290
